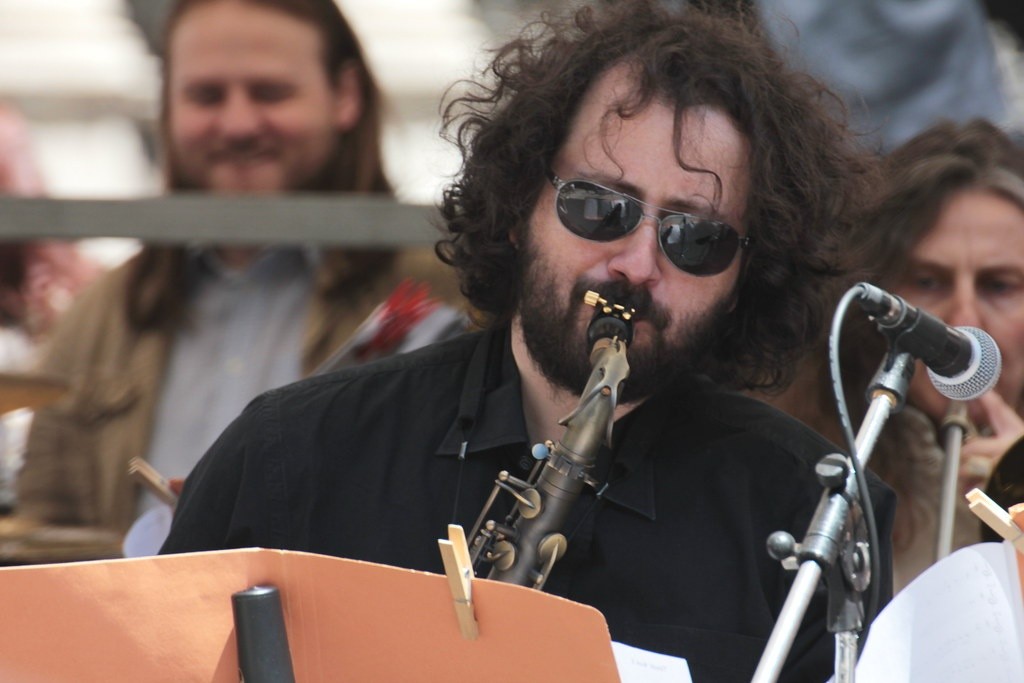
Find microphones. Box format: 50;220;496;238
856;281;1001;400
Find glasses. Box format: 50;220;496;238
545;165;752;277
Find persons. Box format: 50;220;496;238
16;0;482;587
728;0;1024;683
158;0;895;683
0;102;96;509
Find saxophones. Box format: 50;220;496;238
466;288;636;592
